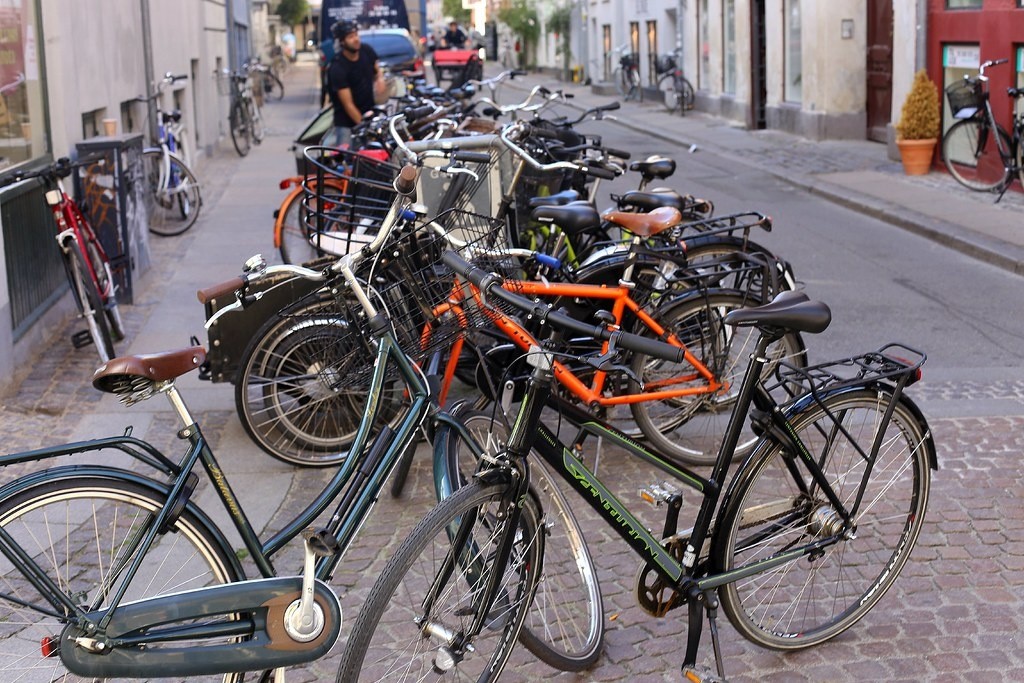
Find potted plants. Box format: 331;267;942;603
895;70;941;175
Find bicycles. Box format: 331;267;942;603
240;54;286;103
212;62;265;157
333;248;938;683
653;46;695;117
0;150;607;683
940;57;1024;204
122;72;203;236
0;151;132;363
606;43;644;104
192;44;811;471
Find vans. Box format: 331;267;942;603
321;0;412;103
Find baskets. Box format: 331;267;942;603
654;53;675;74
620;52;640;69
946;80;983;119
301;144;404;256
278;208;524;389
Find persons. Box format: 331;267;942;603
319;23;338;108
440;19;473;49
328;18;386;152
425;16;483;52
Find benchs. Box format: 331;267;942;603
432;50;482;70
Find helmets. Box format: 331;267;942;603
330;19;358;39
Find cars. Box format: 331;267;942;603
324;28;427;91
